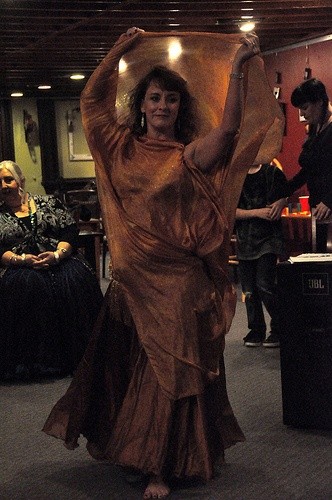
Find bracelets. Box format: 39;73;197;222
54;252;59;262
231;73;244;79
9;254;17;262
60;248;67;254
21;253;25;261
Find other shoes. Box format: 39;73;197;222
243;329;266;346
262;331;279;347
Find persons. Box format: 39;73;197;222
0;160;104;358
41;27;260;500
234;162;289;347
278;79;332;254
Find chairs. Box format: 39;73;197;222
311;207;332;253
65;189;109;280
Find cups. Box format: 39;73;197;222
299;196;310;213
